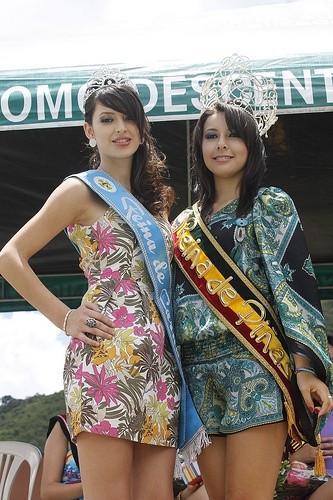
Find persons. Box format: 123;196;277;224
171;101;333;499
0;83;187;499
40;335;333;500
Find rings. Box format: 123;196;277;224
328;396;332;398
86;317;97;328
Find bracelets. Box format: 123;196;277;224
63;310;73;336
295;367;317;376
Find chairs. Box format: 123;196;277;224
0;441;42;500
